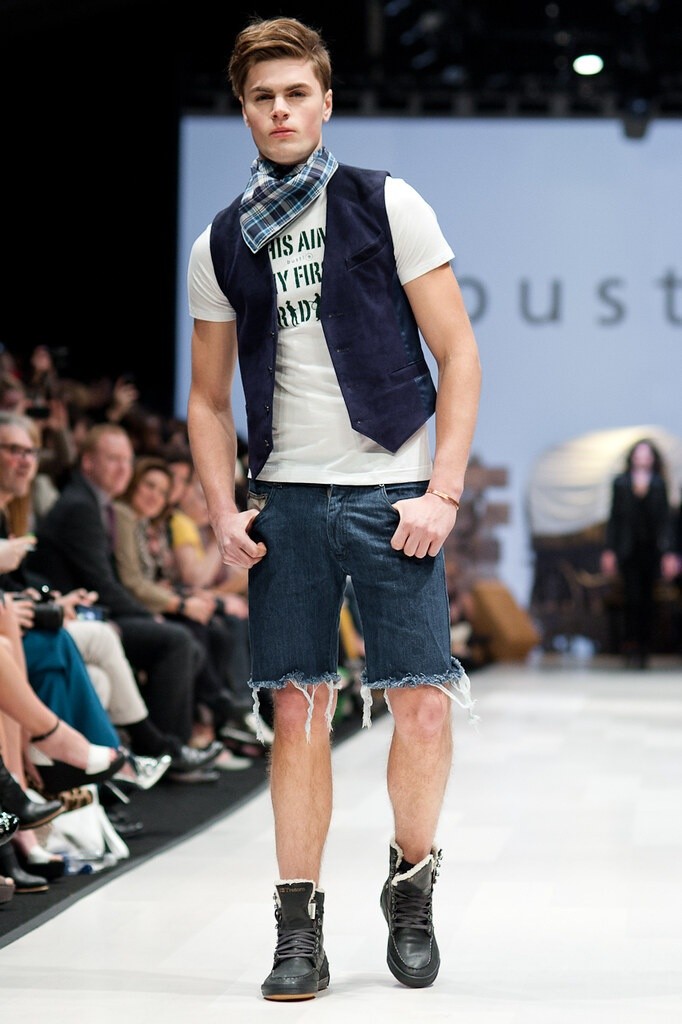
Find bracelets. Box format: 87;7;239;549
425;487;461;511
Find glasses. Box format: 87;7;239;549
0;442;39;457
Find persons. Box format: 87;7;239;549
0;333;682;905
183;16;488;1007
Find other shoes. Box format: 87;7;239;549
0;715;275;905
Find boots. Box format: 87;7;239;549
380;839;443;987
262;879;330;999
